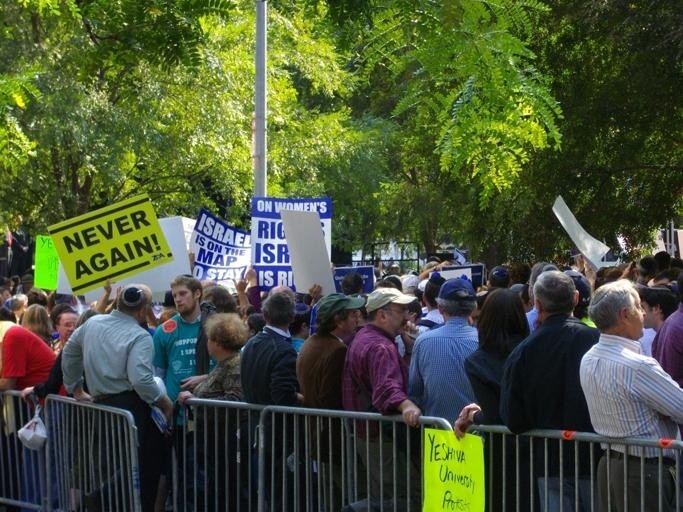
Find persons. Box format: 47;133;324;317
3;253;682;511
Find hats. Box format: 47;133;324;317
564;270;592;304
365;288;417;313
315;293;364;324
437;277;477;301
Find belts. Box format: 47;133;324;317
602;448;676;466
